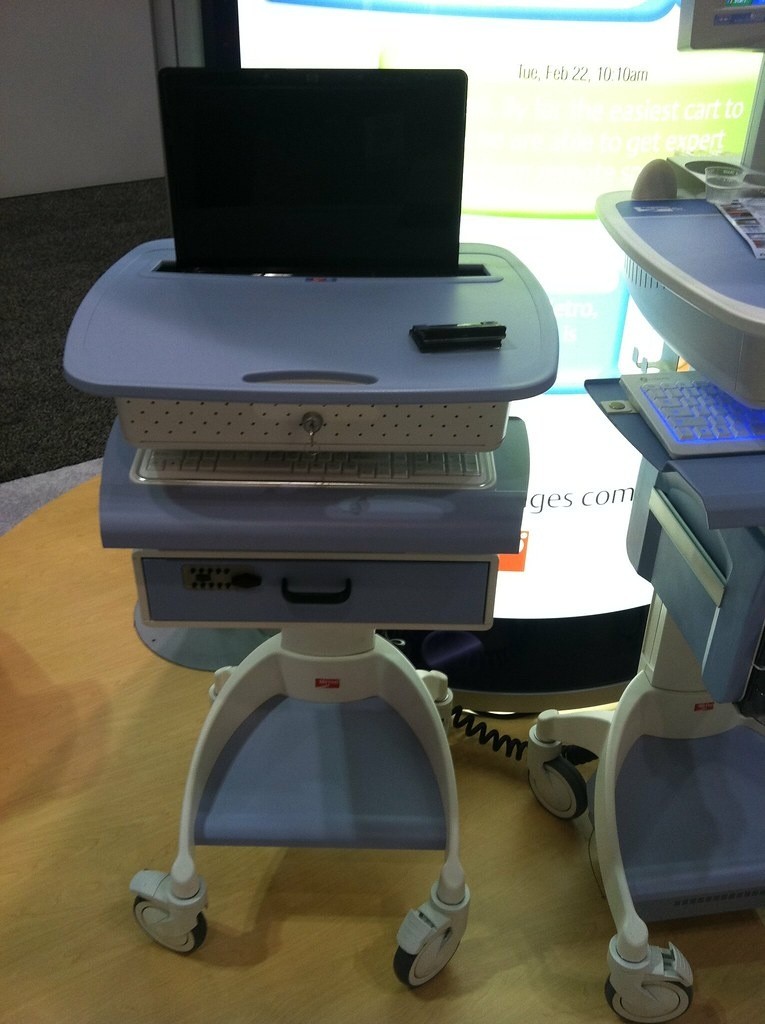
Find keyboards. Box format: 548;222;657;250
128;447;497;491
619;370;765;460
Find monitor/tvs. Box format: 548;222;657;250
676;0;765;53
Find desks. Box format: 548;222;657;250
0;474;765;1024
